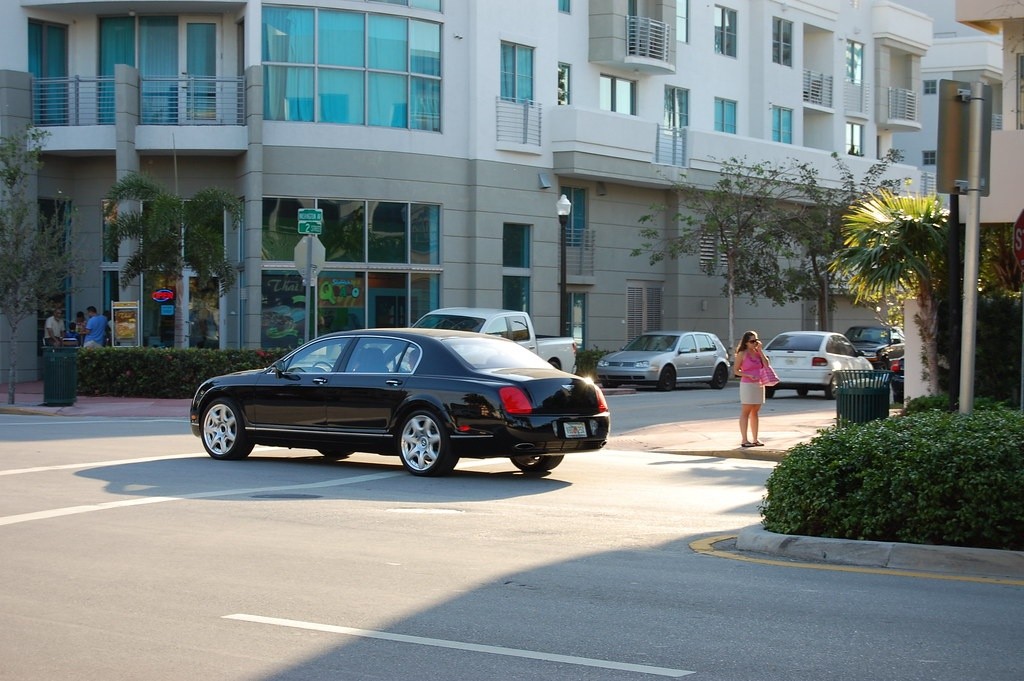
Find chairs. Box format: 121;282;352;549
43;337;60;347
62;337;79;347
355;347;389;372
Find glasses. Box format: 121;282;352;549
747;339;758;344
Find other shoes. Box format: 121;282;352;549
753;441;765;446
741;442;754;447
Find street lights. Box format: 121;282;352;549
555;193;572;337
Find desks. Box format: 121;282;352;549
75;331;89;347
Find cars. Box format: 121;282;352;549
843;325;905;372
595;330;730;393
761;330;874;400
191;329;612;479
888;356;905;404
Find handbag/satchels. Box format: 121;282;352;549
760;359;780;387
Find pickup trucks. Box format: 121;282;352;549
383;307;578;376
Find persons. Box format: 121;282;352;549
44;308;65;347
408;350;416;369
198;292;211;339
64;322;81;345
733;330;769;447
75;310;112;346
79;306;108;347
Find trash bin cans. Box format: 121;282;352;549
836;368;894;427
40;345;80;407
